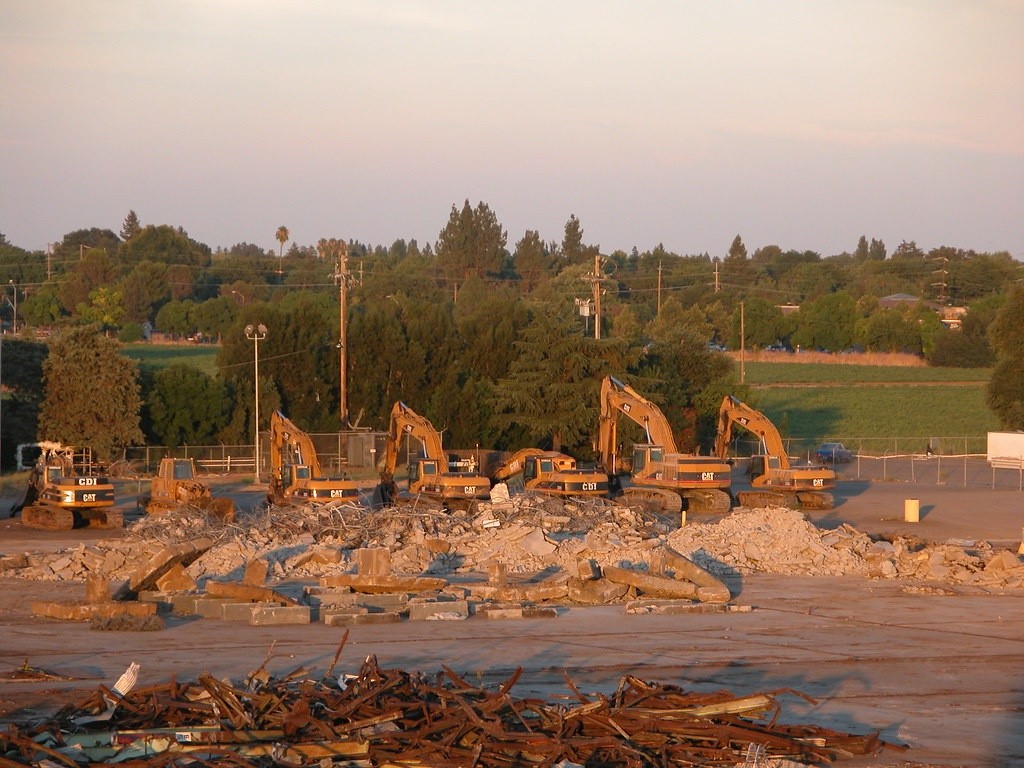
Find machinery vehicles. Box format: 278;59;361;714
378;402;491;511
593;374;735;521
137;457;236;525
16;441;125;531
706;395;837;512
491;448;611;500
267;411;384;507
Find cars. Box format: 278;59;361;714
815;442;852;463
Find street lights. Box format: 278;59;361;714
574;297;595;338
9;279;17;335
244;323;268;484
